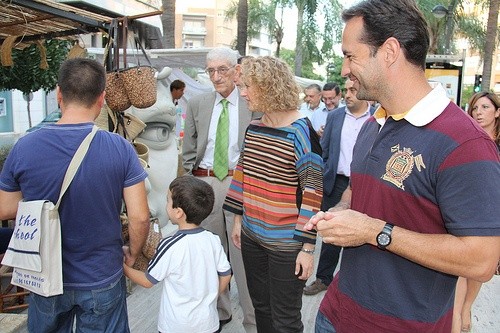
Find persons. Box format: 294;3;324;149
308;0;500;333
158;42;385;333
0;55;152;333
122;176;232;333
450;87;500;333
221;56;324;333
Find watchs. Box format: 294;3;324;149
376;223;394;251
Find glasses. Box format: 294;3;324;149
206;65;234;77
321;94;338;101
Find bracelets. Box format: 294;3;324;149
300;249;315;254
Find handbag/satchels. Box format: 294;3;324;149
116;112;147;142
97;17;131;111
132;142;152;194
119;16;159;109
66;43;88;59
120;214;163;273
1;200;67;297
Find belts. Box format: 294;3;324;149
191;167;234;178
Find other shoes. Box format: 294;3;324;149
303;278;329;295
460;309;471;333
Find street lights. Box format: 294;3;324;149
431;0;458;57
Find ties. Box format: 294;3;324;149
213;99;231;181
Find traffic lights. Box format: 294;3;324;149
472;74;483;95
